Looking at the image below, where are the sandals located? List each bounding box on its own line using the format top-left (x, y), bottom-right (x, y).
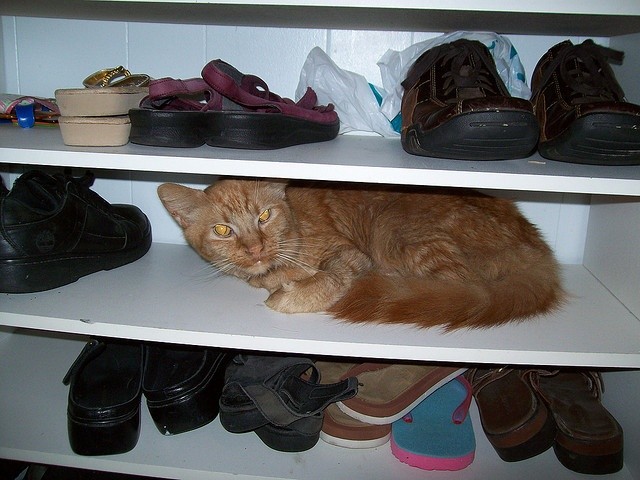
top-left (219, 351), bottom-right (358, 434)
top-left (254, 353), bottom-right (325, 453)
top-left (202, 58), bottom-right (341, 149)
top-left (128, 77), bottom-right (222, 146)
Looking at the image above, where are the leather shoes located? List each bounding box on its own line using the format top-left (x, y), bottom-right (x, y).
top-left (529, 39), bottom-right (640, 164)
top-left (399, 38), bottom-right (540, 161)
top-left (0, 169), bottom-right (152, 293)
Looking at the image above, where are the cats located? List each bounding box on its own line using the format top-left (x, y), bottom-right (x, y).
top-left (157, 173), bottom-right (581, 337)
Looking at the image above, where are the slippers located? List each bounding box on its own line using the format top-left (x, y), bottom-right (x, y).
top-left (315, 357), bottom-right (391, 449)
top-left (335, 363), bottom-right (468, 425)
top-left (390, 375), bottom-right (475, 471)
top-left (55, 65), bottom-right (154, 116)
top-left (142, 341), bottom-right (232, 437)
top-left (535, 364), bottom-right (623, 475)
top-left (467, 363), bottom-right (557, 462)
top-left (63, 335), bottom-right (150, 455)
top-left (59, 118), bottom-right (132, 148)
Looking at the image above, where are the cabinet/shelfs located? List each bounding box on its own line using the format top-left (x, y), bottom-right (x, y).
top-left (0, 0), bottom-right (640, 479)
